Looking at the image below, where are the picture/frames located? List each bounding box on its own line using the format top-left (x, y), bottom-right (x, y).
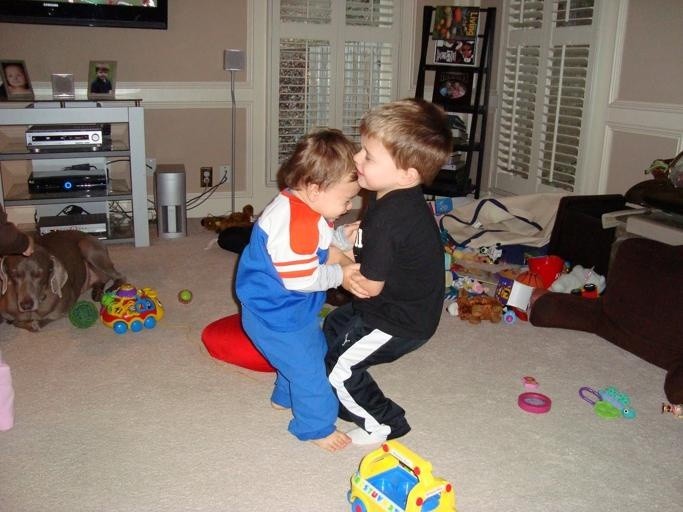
top-left (433, 38), bottom-right (479, 67)
top-left (432, 69), bottom-right (474, 108)
top-left (88, 61), bottom-right (116, 101)
top-left (0, 60), bottom-right (34, 101)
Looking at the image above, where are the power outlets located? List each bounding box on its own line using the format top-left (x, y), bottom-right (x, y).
top-left (201, 167), bottom-right (212, 187)
top-left (219, 165), bottom-right (232, 183)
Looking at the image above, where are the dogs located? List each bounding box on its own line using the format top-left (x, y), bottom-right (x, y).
top-left (0, 230), bottom-right (127, 333)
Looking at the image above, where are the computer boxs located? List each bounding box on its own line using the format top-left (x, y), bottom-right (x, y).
top-left (156, 163), bottom-right (187, 239)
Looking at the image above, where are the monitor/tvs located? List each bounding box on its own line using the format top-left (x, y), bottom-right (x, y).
top-left (0, 0), bottom-right (168, 30)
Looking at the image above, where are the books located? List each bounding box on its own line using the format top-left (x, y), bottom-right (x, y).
top-left (431, 3), bottom-right (480, 112)
top-left (448, 114), bottom-right (471, 145)
top-left (443, 154), bottom-right (466, 171)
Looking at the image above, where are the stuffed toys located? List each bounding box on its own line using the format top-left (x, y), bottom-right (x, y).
top-left (446, 291), bottom-right (505, 325)
top-left (201, 204), bottom-right (256, 233)
top-left (548, 267), bottom-right (609, 297)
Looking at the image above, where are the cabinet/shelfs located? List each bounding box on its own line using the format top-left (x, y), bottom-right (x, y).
top-left (414, 5), bottom-right (497, 199)
top-left (0, 101), bottom-right (150, 247)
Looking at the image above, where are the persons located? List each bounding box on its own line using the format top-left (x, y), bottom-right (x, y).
top-left (91, 65), bottom-right (112, 93)
top-left (5, 62), bottom-right (28, 93)
top-left (320, 98), bottom-right (452, 446)
top-left (233, 128), bottom-right (372, 452)
top-left (0, 202), bottom-right (35, 256)
